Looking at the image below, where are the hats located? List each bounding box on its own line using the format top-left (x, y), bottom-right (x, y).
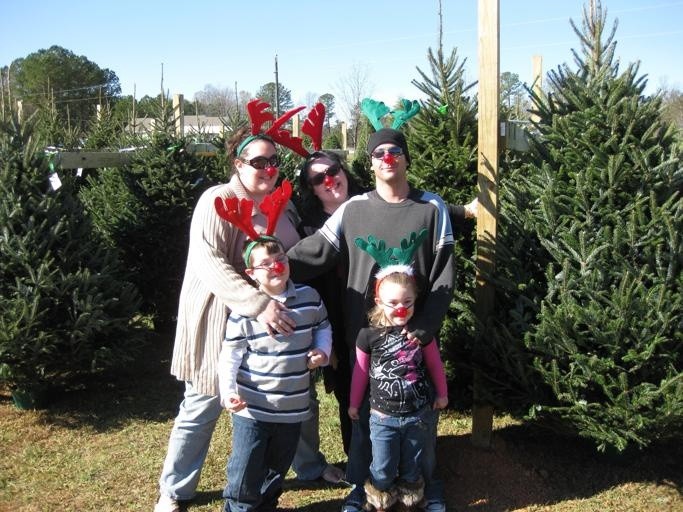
top-left (367, 127), bottom-right (410, 157)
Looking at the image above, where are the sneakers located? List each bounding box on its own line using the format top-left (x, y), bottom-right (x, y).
top-left (154, 496), bottom-right (181, 512)
top-left (341, 481), bottom-right (375, 512)
top-left (420, 487), bottom-right (446, 511)
top-left (322, 461), bottom-right (347, 480)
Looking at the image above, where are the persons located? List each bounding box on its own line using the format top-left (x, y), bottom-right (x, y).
top-left (218, 233), bottom-right (334, 512)
top-left (349, 263), bottom-right (450, 511)
top-left (287, 130), bottom-right (456, 511)
top-left (157, 127), bottom-right (346, 512)
top-left (294, 147), bottom-right (481, 457)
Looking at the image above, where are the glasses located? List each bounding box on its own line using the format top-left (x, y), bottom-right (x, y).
top-left (239, 154), bottom-right (282, 170)
top-left (371, 147), bottom-right (403, 158)
top-left (308, 162), bottom-right (342, 186)
top-left (253, 254), bottom-right (290, 270)
